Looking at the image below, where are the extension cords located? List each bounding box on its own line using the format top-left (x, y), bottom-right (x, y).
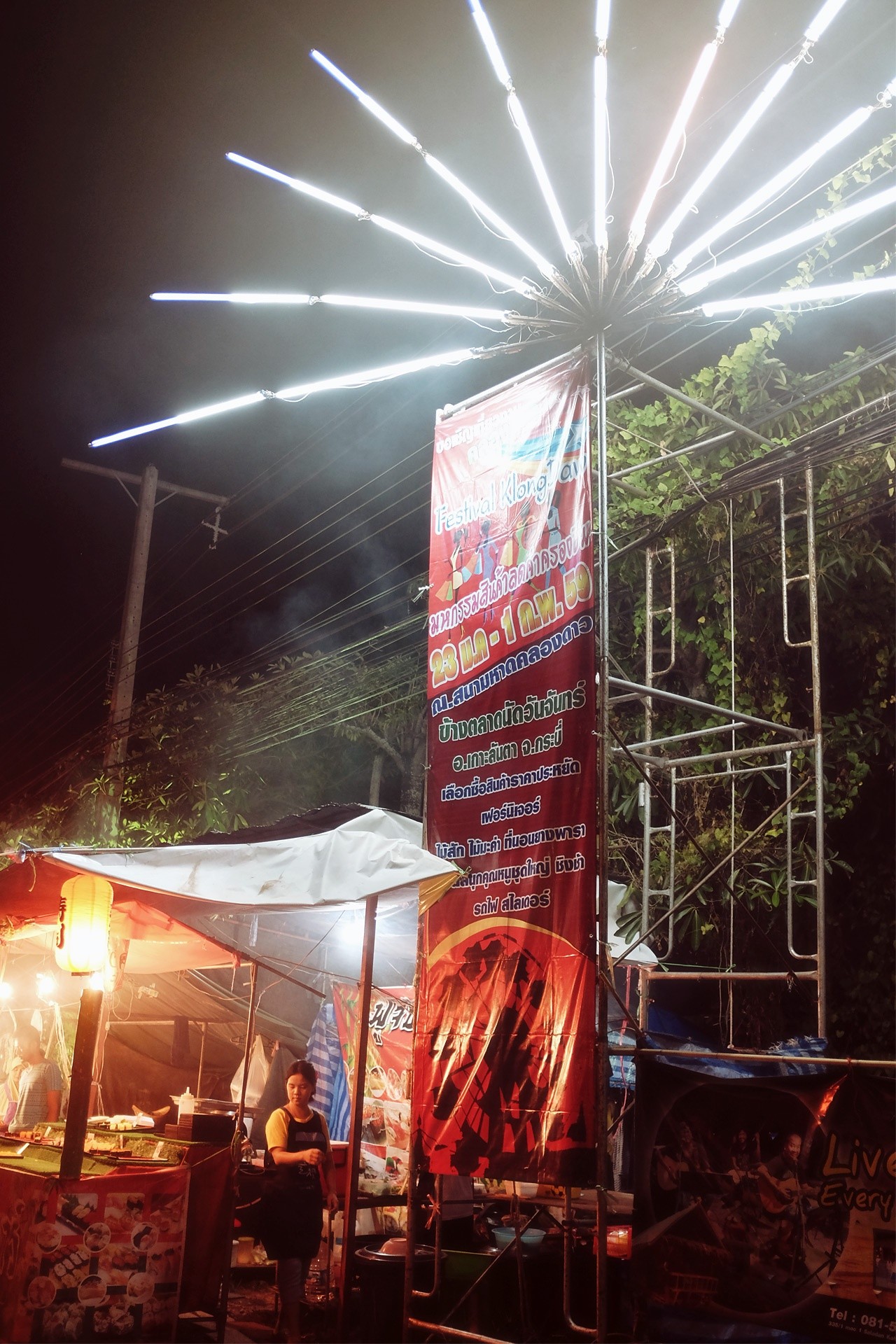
top-left (138, 986), bottom-right (159, 997)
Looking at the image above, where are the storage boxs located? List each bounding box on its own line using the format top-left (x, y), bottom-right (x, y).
top-left (318, 1140), bottom-right (349, 1199)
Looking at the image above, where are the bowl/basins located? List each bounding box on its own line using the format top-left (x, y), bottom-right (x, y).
top-left (131, 1105), bottom-right (171, 1126)
top-left (515, 1182), bottom-right (538, 1198)
top-left (378, 1237), bottom-right (407, 1255)
top-left (491, 1227), bottom-right (546, 1249)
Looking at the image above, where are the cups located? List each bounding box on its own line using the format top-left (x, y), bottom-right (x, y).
top-left (237, 1237), bottom-right (254, 1264)
top-left (230, 1240), bottom-right (239, 1268)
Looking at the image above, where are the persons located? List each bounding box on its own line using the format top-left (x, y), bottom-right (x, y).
top-left (253, 1059), bottom-right (339, 1344)
top-left (8, 1024), bottom-right (63, 1132)
top-left (637, 1064), bottom-right (821, 1308)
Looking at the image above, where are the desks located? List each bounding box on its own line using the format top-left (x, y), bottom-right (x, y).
top-left (476, 1184), bottom-right (635, 1344)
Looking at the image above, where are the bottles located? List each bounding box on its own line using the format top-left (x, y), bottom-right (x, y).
top-left (304, 1241), bottom-right (336, 1304)
top-left (177, 1086), bottom-right (195, 1125)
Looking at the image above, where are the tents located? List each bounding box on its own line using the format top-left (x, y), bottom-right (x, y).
top-left (0, 803), bottom-right (656, 1344)
top-left (304, 1006), bottom-right (830, 1207)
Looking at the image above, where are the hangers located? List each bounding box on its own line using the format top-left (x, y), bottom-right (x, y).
top-left (230, 1021), bottom-right (280, 1048)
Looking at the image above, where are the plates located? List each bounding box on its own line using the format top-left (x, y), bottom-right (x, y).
top-left (77, 1274), bottom-right (107, 1307)
top-left (343, 1060), bottom-right (406, 1101)
top-left (126, 1272), bottom-right (154, 1304)
top-left (131, 1221), bottom-right (159, 1252)
top-left (27, 1276), bottom-right (57, 1309)
top-left (83, 1223), bottom-right (111, 1253)
top-left (35, 1222), bottom-right (62, 1254)
